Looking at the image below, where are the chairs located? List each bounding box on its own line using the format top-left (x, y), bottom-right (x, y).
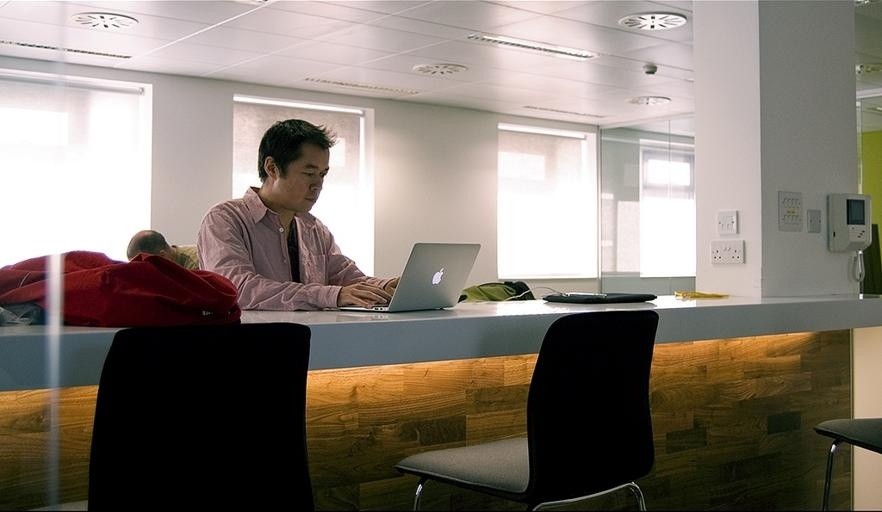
top-left (813, 418), bottom-right (882, 512)
top-left (88, 322), bottom-right (315, 512)
top-left (395, 310), bottom-right (659, 512)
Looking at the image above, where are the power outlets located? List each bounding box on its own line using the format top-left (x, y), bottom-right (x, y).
top-left (710, 239), bottom-right (726, 264)
top-left (726, 240), bottom-right (745, 266)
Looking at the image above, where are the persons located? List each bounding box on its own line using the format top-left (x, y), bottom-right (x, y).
top-left (197, 118), bottom-right (401, 313)
top-left (127, 230), bottom-right (201, 271)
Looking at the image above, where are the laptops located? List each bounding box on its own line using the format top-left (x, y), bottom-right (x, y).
top-left (338, 244), bottom-right (482, 311)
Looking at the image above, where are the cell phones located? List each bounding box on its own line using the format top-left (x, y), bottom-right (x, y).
top-left (565, 293), bottom-right (608, 297)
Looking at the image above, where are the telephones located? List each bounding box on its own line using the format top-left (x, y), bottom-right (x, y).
top-left (828, 193), bottom-right (872, 253)
